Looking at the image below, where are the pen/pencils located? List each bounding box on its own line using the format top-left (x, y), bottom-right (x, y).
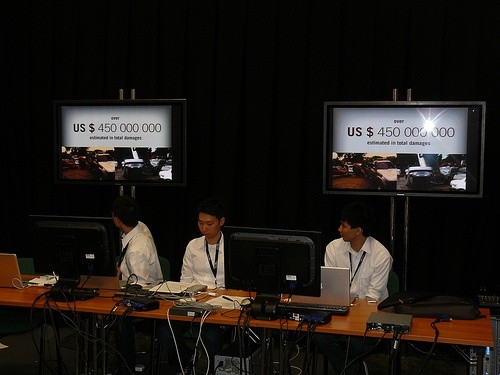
top-left (222, 295), bottom-right (234, 301)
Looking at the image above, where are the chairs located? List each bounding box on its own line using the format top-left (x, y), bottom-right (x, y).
top-left (117, 257), bottom-right (170, 375)
top-left (324, 272), bottom-right (403, 375)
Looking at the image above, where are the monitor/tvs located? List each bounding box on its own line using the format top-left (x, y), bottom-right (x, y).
top-left (223, 226), bottom-right (322, 320)
top-left (50, 99), bottom-right (187, 187)
top-left (27, 214), bottom-right (120, 300)
top-left (322, 101), bottom-right (486, 198)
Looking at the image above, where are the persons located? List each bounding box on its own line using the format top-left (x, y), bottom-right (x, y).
top-left (398, 175), bottom-right (408, 190)
top-left (312, 202), bottom-right (393, 375)
top-left (78, 197), bottom-right (164, 375)
top-left (115, 167), bottom-right (124, 180)
top-left (168, 204), bottom-right (226, 375)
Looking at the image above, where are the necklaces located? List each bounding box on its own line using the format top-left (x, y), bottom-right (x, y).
top-left (116, 233), bottom-right (130, 270)
top-left (204, 237), bottom-right (221, 284)
top-left (348, 251), bottom-right (366, 287)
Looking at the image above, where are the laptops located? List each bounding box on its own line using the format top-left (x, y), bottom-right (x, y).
top-left (0, 253), bottom-right (34, 288)
top-left (79, 262), bottom-right (126, 290)
top-left (291, 267), bottom-right (358, 306)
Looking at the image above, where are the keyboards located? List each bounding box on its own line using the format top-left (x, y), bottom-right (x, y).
top-left (281, 302), bottom-right (349, 315)
top-left (377, 292), bottom-right (422, 311)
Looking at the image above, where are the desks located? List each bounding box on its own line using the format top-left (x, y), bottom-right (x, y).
top-left (0, 273), bottom-right (493, 375)
